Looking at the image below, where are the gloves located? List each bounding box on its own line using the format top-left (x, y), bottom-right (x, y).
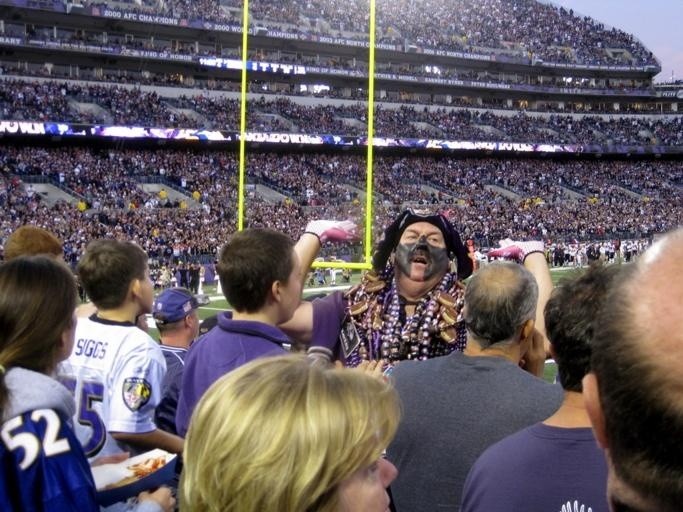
top-left (305, 219), bottom-right (358, 247)
top-left (486, 238), bottom-right (545, 264)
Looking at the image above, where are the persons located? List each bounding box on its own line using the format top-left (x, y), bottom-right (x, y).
top-left (378, 259), bottom-right (566, 512)
top-left (73, 298), bottom-right (149, 335)
top-left (1, 255), bottom-right (176, 510)
top-left (178, 355), bottom-right (403, 510)
top-left (176, 226), bottom-right (304, 438)
top-left (49, 238), bottom-right (185, 454)
top-left (1, 1), bottom-right (681, 296)
top-left (460, 262), bottom-right (618, 511)
top-left (151, 286), bottom-right (200, 436)
top-left (580, 224), bottom-right (681, 511)
top-left (2, 225), bottom-right (65, 264)
top-left (275, 208), bottom-right (555, 382)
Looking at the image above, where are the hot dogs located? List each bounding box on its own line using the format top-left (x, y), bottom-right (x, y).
top-left (105, 456), bottom-right (166, 490)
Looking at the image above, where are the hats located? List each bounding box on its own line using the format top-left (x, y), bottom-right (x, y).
top-left (151, 287), bottom-right (210, 324)
